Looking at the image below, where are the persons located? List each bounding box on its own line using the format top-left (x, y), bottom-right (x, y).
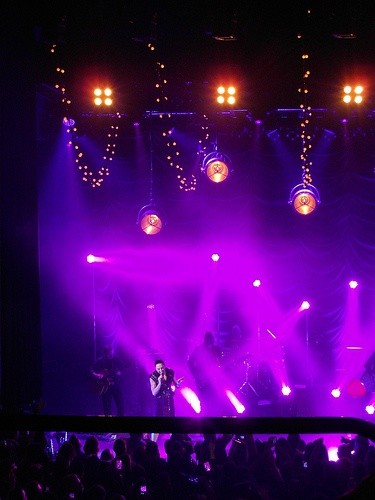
top-left (188, 332), bottom-right (227, 416)
top-left (148, 360), bottom-right (186, 417)
top-left (0, 428), bottom-right (375, 500)
top-left (241, 351), bottom-right (279, 411)
top-left (90, 346), bottom-right (125, 416)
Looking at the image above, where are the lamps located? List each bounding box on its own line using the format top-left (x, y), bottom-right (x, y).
top-left (288, 184), bottom-right (321, 216)
top-left (201, 151), bottom-right (234, 182)
top-left (136, 204), bottom-right (165, 237)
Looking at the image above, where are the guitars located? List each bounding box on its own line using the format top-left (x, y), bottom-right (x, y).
top-left (93, 362), bottom-right (129, 396)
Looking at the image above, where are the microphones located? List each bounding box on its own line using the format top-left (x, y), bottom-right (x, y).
top-left (160, 372), bottom-right (164, 383)
